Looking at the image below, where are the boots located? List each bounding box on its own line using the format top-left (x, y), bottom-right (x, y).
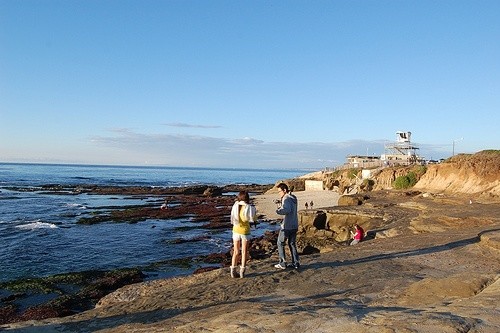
top-left (229, 266), bottom-right (238, 278)
top-left (239, 266), bottom-right (246, 278)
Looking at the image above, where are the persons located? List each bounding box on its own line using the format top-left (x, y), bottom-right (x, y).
top-left (230, 191), bottom-right (258, 279)
top-left (349, 224), bottom-right (363, 246)
top-left (310, 201), bottom-right (314, 210)
top-left (160, 199), bottom-right (171, 212)
top-left (273, 183), bottom-right (301, 271)
top-left (305, 202), bottom-right (308, 210)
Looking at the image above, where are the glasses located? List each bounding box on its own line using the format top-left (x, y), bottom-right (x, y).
top-left (278, 190), bottom-right (282, 193)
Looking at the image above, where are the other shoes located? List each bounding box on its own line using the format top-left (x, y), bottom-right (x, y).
top-left (275, 264), bottom-right (286, 270)
top-left (287, 264), bottom-right (298, 269)
